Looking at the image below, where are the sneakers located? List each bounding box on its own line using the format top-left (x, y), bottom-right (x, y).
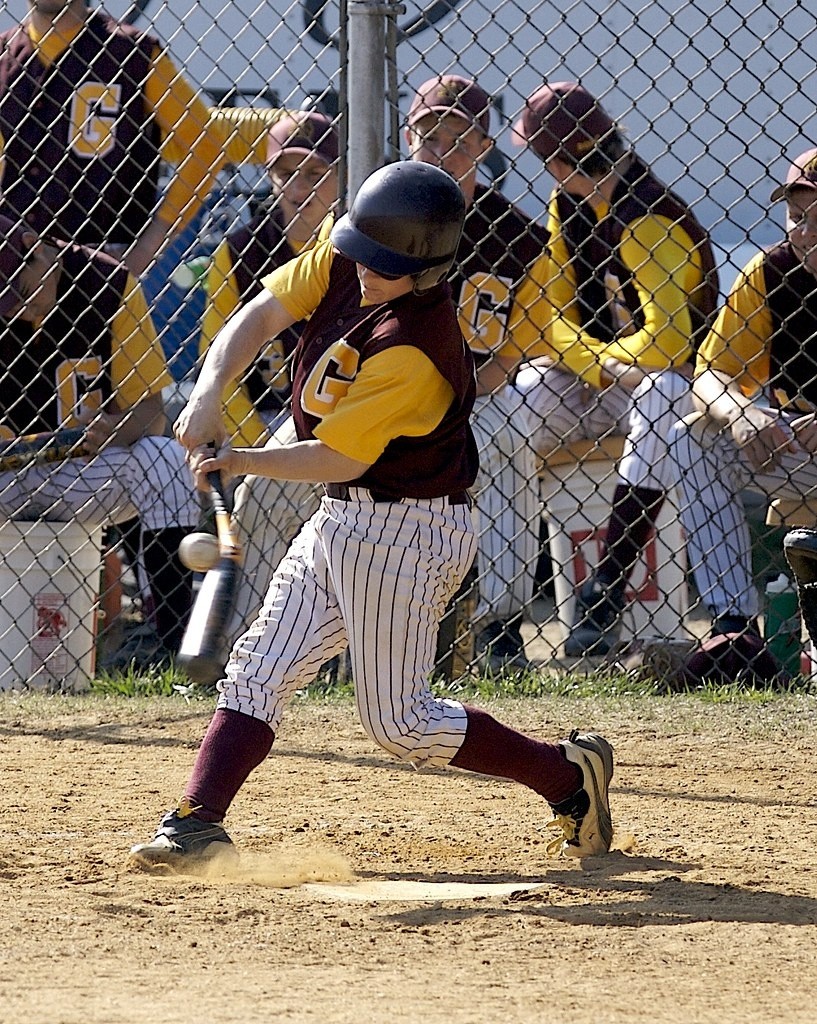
top-left (129, 795), bottom-right (240, 876)
top-left (564, 577), bottom-right (626, 656)
top-left (471, 611), bottom-right (539, 684)
top-left (538, 729), bottom-right (613, 858)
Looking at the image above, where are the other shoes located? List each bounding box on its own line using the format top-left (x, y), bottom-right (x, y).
top-left (102, 624), bottom-right (171, 679)
top-left (295, 655), bottom-right (340, 697)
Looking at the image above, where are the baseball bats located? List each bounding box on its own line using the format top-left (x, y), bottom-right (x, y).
top-left (177, 443), bottom-right (243, 686)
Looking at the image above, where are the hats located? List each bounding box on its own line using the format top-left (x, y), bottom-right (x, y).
top-left (0, 215), bottom-right (26, 317)
top-left (263, 113), bottom-right (339, 166)
top-left (770, 148), bottom-right (817, 204)
top-left (512, 81), bottom-right (613, 157)
top-left (406, 75), bottom-right (490, 136)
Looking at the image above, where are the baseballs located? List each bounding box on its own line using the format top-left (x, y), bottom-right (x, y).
top-left (177, 532), bottom-right (220, 573)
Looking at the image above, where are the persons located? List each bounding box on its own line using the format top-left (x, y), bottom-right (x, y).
top-left (0, 0), bottom-right (228, 283)
top-left (661, 147), bottom-right (817, 692)
top-left (508, 80), bottom-right (720, 655)
top-left (127, 162), bottom-right (616, 882)
top-left (199, 112), bottom-right (343, 529)
top-left (214, 74), bottom-right (553, 681)
top-left (0, 215), bottom-right (199, 681)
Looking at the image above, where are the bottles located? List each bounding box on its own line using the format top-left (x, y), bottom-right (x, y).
top-left (173, 256), bottom-right (211, 289)
top-left (762, 574), bottom-right (803, 690)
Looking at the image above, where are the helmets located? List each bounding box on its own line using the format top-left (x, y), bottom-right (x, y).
top-left (330, 160), bottom-right (466, 291)
